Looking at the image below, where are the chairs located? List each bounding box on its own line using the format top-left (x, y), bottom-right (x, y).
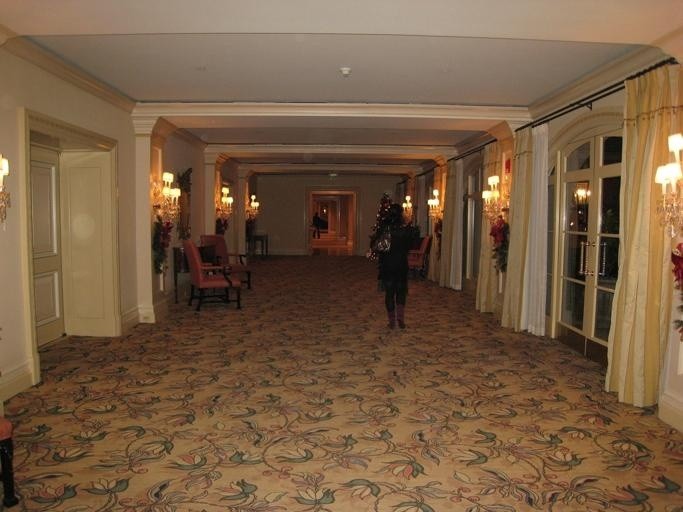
top-left (404, 236), bottom-right (430, 278)
top-left (184, 232), bottom-right (254, 311)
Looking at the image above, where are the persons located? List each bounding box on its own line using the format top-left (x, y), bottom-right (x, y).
top-left (370, 204), bottom-right (424, 329)
top-left (313, 212), bottom-right (320, 239)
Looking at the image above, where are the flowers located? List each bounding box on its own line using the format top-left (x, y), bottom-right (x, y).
top-left (489, 220), bottom-right (511, 272)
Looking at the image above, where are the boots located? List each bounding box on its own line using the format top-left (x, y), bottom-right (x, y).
top-left (386, 309), bottom-right (395, 330)
top-left (396, 304), bottom-right (406, 329)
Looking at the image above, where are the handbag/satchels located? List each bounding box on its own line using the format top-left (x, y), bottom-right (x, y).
top-left (371, 231), bottom-right (391, 252)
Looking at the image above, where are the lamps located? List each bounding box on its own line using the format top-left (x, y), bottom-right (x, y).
top-left (655, 132), bottom-right (683, 242)
top-left (428, 190), bottom-right (444, 222)
top-left (245, 194), bottom-right (261, 221)
top-left (158, 172), bottom-right (183, 222)
top-left (477, 174), bottom-right (513, 224)
top-left (216, 186), bottom-right (232, 229)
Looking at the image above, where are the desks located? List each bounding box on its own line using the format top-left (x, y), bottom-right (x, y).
top-left (174, 245), bottom-right (218, 304)
top-left (247, 232), bottom-right (270, 261)
